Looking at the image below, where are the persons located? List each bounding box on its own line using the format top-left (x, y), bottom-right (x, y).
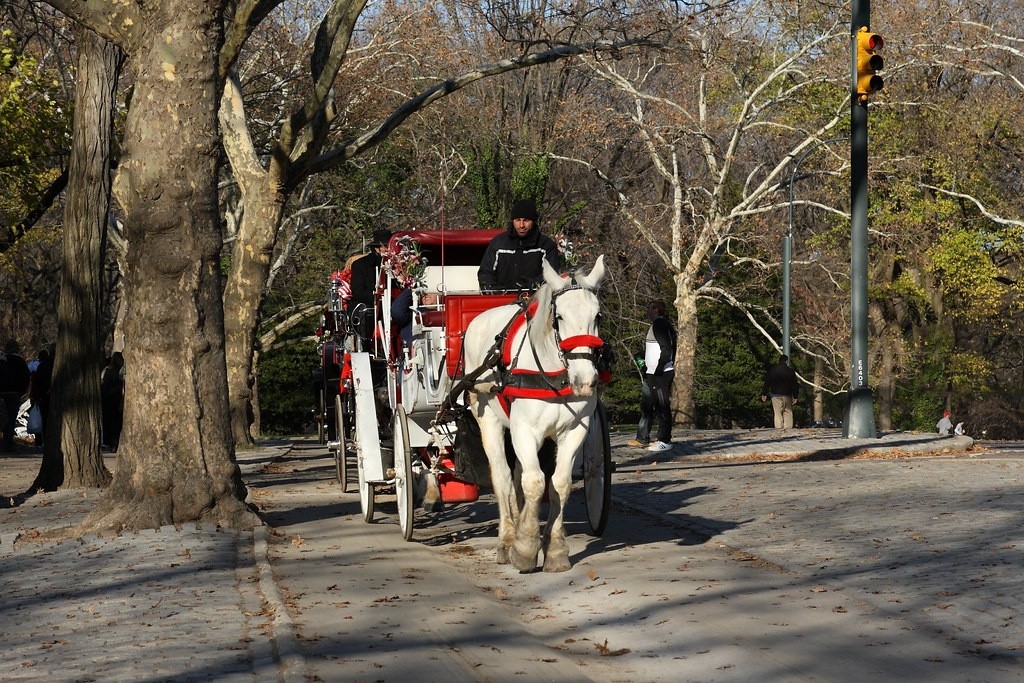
top-left (477, 200), bottom-right (566, 295)
top-left (391, 284), bottom-right (443, 384)
top-left (627, 300), bottom-right (677, 452)
top-left (762, 354), bottom-right (798, 429)
top-left (346, 229), bottom-right (392, 340)
top-left (0, 339), bottom-right (124, 454)
top-left (936, 411), bottom-right (965, 436)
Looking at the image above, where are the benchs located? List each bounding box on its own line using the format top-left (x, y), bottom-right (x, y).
top-left (408, 265), bottom-right (485, 382)
top-left (317, 300), bottom-right (397, 435)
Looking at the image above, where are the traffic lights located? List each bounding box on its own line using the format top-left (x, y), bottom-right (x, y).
top-left (850, 25), bottom-right (884, 105)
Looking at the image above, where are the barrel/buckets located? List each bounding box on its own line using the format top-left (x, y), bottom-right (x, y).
top-left (437, 455), bottom-right (478, 503)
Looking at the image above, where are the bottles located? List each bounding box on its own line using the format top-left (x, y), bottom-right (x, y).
top-left (636, 357), bottom-right (647, 373)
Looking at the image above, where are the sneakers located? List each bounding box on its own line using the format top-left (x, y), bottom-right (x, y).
top-left (648, 441), bottom-right (673, 452)
top-left (627, 440), bottom-right (649, 447)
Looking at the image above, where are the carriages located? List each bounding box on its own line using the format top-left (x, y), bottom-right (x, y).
top-left (313, 229), bottom-right (618, 576)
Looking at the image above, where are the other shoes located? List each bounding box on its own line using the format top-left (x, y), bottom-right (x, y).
top-left (2, 441), bottom-right (15, 447)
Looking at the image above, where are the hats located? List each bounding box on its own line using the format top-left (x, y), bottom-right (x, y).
top-left (368, 230), bottom-right (392, 246)
top-left (510, 200), bottom-right (538, 222)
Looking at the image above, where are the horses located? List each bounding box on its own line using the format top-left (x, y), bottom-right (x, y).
top-left (422, 454), bottom-right (446, 511)
top-left (463, 255), bottom-right (606, 573)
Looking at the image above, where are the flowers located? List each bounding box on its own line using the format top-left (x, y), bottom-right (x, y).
top-left (379, 235), bottom-right (428, 289)
